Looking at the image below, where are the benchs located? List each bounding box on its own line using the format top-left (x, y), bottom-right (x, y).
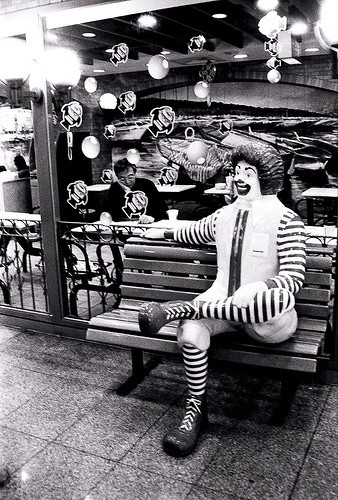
top-left (86, 236), bottom-right (334, 426)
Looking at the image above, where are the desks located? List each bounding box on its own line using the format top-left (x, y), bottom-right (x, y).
top-left (88, 183), bottom-right (196, 209)
top-left (302, 186), bottom-right (338, 226)
top-left (204, 185), bottom-right (284, 197)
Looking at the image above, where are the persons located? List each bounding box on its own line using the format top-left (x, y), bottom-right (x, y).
top-left (97, 158), bottom-right (170, 224)
top-left (138, 141), bottom-right (307, 458)
top-left (14, 154), bottom-right (30, 178)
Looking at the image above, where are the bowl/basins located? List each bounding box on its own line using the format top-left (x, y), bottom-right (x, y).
top-left (215, 183), bottom-right (226, 190)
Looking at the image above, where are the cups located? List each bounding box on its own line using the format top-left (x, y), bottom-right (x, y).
top-left (225, 176), bottom-right (233, 187)
top-left (167, 209), bottom-right (178, 220)
